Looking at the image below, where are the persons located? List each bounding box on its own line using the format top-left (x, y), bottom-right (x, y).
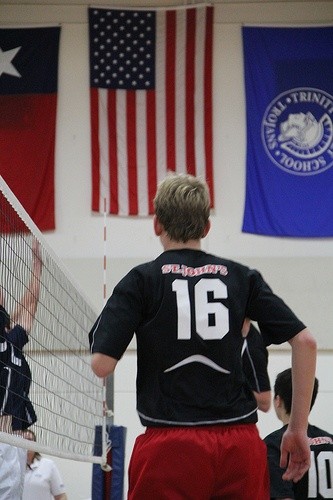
top-left (263, 368), bottom-right (333, 500)
top-left (88, 174), bottom-right (316, 500)
top-left (241, 319), bottom-right (272, 413)
top-left (21, 430), bottom-right (67, 500)
top-left (0, 236), bottom-right (42, 500)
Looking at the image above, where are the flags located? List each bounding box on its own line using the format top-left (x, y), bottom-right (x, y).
top-left (0, 22), bottom-right (61, 235)
top-left (241, 23), bottom-right (333, 240)
top-left (88, 4), bottom-right (216, 216)
